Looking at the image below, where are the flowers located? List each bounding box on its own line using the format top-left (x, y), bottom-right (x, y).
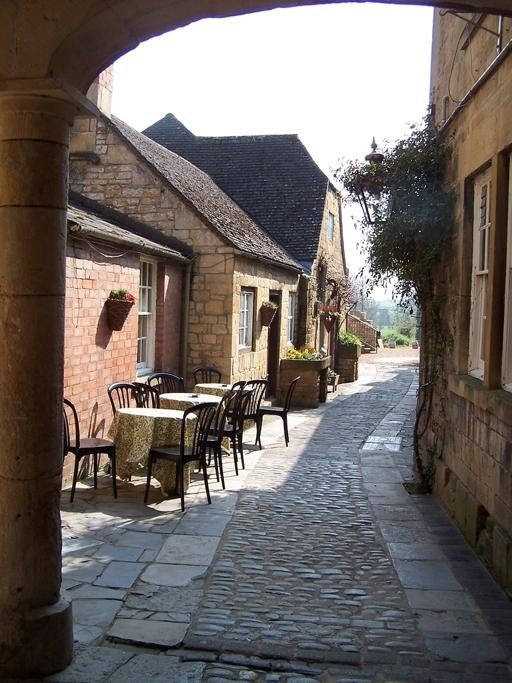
top-left (109, 287), bottom-right (135, 302)
top-left (262, 299), bottom-right (278, 309)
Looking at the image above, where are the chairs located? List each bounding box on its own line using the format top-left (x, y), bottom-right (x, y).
top-left (62, 366), bottom-right (305, 512)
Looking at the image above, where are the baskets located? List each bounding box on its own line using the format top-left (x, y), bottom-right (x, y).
top-left (106, 298), bottom-right (134, 330)
top-left (261, 307), bottom-right (277, 326)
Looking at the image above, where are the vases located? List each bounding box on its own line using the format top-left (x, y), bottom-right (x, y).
top-left (260, 306), bottom-right (279, 327)
top-left (102, 298), bottom-right (136, 332)
